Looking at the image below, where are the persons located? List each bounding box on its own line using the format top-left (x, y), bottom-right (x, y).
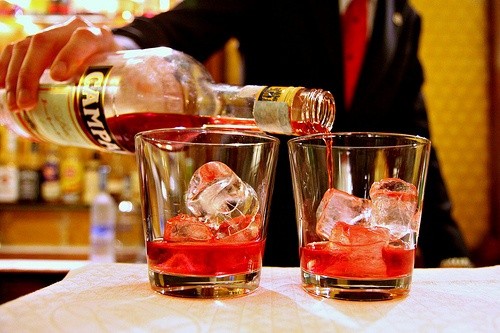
top-left (0, 0), bottom-right (474, 267)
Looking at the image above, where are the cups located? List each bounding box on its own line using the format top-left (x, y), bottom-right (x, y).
top-left (134, 128), bottom-right (279, 300)
top-left (287, 132), bottom-right (431, 301)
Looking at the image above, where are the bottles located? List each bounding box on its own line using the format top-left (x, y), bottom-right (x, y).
top-left (0, 45), bottom-right (336, 154)
top-left (0, 133), bottom-right (102, 206)
top-left (88, 166), bottom-right (116, 264)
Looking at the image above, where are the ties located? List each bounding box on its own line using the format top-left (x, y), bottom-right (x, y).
top-left (341, 0), bottom-right (368, 111)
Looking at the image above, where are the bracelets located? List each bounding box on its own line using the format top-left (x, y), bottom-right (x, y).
top-left (438, 259), bottom-right (470, 266)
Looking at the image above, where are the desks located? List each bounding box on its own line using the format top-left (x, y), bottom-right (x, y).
top-left (0, 263), bottom-right (500, 333)
